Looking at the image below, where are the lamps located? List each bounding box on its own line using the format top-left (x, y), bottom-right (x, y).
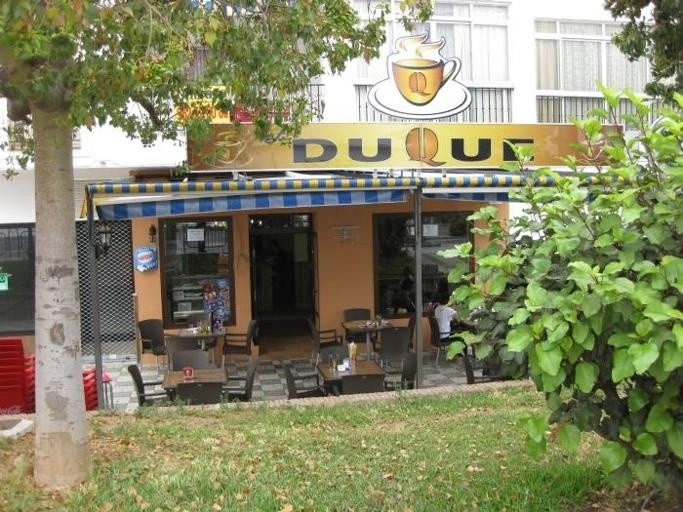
top-left (94, 221), bottom-right (117, 257)
top-left (405, 219), bottom-right (416, 242)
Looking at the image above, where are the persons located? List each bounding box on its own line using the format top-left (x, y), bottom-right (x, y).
top-left (433, 293), bottom-right (469, 357)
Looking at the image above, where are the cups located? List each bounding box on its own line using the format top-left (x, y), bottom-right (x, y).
top-left (337, 358), bottom-right (350, 371)
top-left (392, 57), bottom-right (461, 105)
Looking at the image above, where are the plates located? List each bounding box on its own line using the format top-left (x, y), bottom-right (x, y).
top-left (367, 77), bottom-right (472, 120)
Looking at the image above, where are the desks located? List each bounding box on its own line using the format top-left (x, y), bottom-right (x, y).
top-left (161, 326), bottom-right (227, 361)
top-left (161, 368), bottom-right (230, 404)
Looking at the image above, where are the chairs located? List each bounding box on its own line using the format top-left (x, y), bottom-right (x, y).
top-left (222, 345), bottom-right (261, 402)
top-left (220, 319), bottom-right (259, 370)
top-left (147, 323), bottom-right (174, 373)
top-left (163, 334), bottom-right (205, 353)
top-left (136, 319), bottom-right (165, 355)
top-left (169, 349), bottom-right (209, 372)
top-left (127, 363), bottom-right (174, 406)
top-left (275, 307), bottom-right (490, 397)
top-left (0, 336), bottom-right (113, 414)
top-left (187, 312), bottom-right (208, 324)
top-left (176, 379), bottom-right (223, 405)
